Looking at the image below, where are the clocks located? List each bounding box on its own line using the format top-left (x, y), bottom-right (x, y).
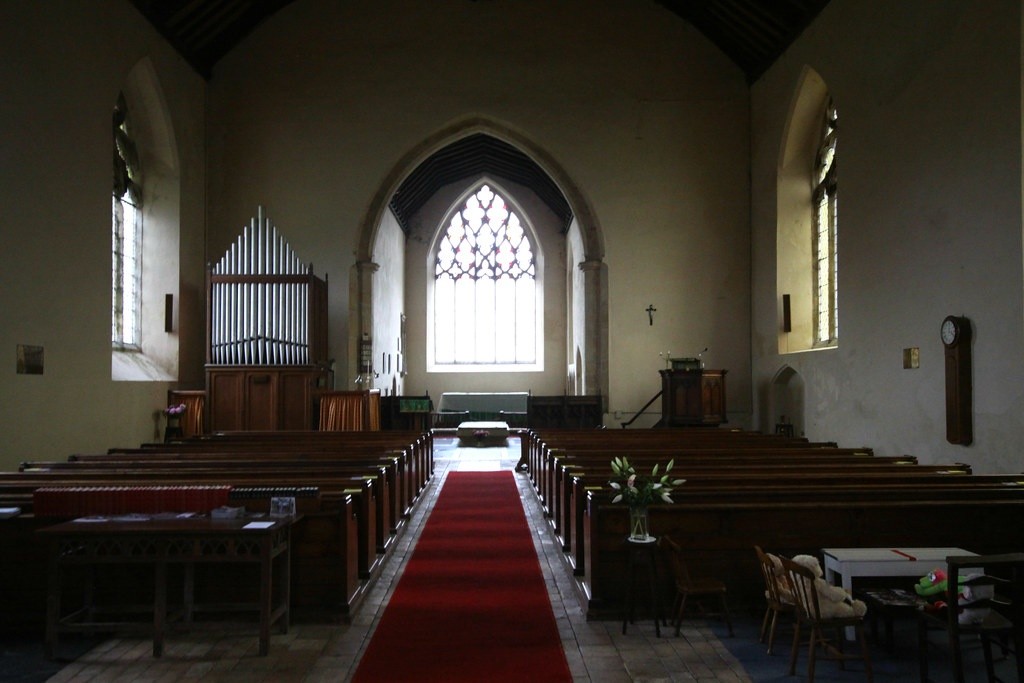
top-left (941, 316), bottom-right (971, 348)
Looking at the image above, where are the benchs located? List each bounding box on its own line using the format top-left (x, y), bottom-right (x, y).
top-left (515, 421), bottom-right (1023, 621)
top-left (0, 433), bottom-right (432, 626)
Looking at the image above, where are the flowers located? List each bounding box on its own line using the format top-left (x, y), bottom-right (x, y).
top-left (162, 402), bottom-right (186, 418)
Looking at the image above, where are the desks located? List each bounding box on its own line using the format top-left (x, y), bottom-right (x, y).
top-left (818, 547), bottom-right (987, 649)
top-left (32, 513), bottom-right (294, 660)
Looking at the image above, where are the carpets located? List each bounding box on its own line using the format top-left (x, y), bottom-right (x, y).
top-left (350, 471), bottom-right (575, 683)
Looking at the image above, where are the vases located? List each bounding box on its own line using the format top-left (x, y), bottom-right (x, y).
top-left (169, 419), bottom-right (180, 428)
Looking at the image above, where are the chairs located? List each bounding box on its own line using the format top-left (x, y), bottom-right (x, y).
top-left (915, 554), bottom-right (1023, 682)
top-left (780, 551), bottom-right (876, 683)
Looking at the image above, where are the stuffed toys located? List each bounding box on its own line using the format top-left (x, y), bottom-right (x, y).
top-left (790, 552), bottom-right (868, 620)
top-left (914, 568), bottom-right (971, 606)
top-left (955, 573), bottom-right (992, 627)
top-left (761, 551), bottom-right (797, 607)
